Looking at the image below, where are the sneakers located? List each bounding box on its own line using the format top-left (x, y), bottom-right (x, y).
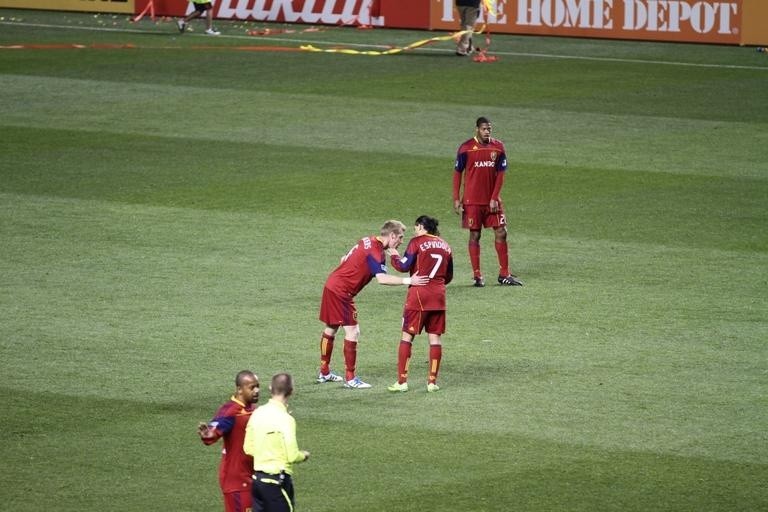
top-left (473, 276), bottom-right (485, 287)
top-left (317, 372), bottom-right (343, 383)
top-left (456, 48), bottom-right (469, 56)
top-left (470, 46), bottom-right (475, 53)
top-left (204, 28), bottom-right (221, 36)
top-left (344, 376), bottom-right (372, 389)
top-left (388, 381), bottom-right (408, 392)
top-left (176, 18), bottom-right (186, 33)
top-left (427, 383), bottom-right (439, 392)
top-left (498, 274), bottom-right (523, 287)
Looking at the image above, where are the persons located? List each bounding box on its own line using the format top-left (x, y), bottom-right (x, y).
top-left (243, 373), bottom-right (310, 512)
top-left (176, 0), bottom-right (221, 35)
top-left (386, 216), bottom-right (453, 393)
top-left (436, 0), bottom-right (508, 57)
top-left (316, 219), bottom-right (430, 390)
top-left (452, 117), bottom-right (524, 287)
top-left (197, 370), bottom-right (260, 512)
